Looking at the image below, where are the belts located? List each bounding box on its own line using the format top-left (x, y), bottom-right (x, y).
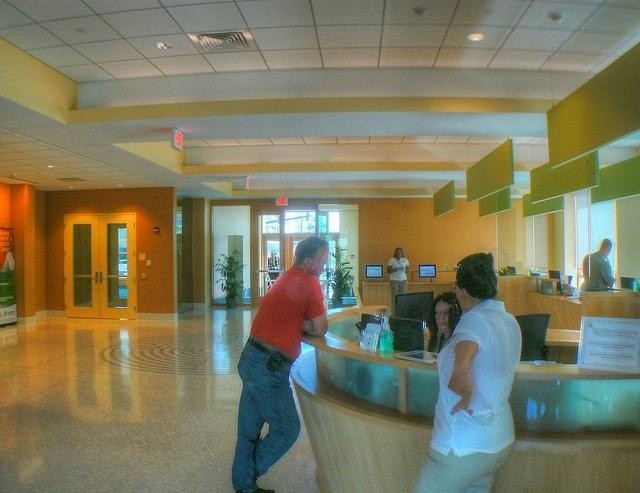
top-left (248, 339), bottom-right (293, 363)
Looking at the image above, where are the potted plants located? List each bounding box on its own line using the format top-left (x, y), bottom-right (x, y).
top-left (215, 250), bottom-right (248, 309)
top-left (325, 244), bottom-right (356, 309)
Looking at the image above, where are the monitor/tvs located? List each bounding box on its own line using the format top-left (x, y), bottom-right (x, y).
top-left (419, 265), bottom-right (436, 278)
top-left (620, 277), bottom-right (640, 290)
top-left (507, 265), bottom-right (516, 273)
top-left (361, 314), bottom-right (423, 351)
top-left (366, 264), bottom-right (383, 279)
top-left (549, 269), bottom-right (561, 292)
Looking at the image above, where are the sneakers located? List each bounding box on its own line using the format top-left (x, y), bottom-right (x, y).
top-left (236, 488), bottom-right (274, 493)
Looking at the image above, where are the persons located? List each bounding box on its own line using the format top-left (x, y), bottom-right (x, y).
top-left (409, 249), bottom-right (524, 492)
top-left (578, 239), bottom-right (616, 294)
top-left (425, 294), bottom-right (463, 356)
top-left (386, 247), bottom-right (411, 316)
top-left (230, 235), bottom-right (329, 493)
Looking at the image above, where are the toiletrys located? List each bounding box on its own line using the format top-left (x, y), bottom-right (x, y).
top-left (379, 316), bottom-right (395, 353)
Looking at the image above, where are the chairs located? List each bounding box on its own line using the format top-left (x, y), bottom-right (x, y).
top-left (513, 313), bottom-right (553, 363)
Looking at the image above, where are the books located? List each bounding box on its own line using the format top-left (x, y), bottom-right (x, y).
top-left (362, 322), bottom-right (380, 351)
top-left (395, 348), bottom-right (441, 366)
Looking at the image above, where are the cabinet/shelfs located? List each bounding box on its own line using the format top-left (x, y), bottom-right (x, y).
top-left (360, 266), bottom-right (640, 333)
